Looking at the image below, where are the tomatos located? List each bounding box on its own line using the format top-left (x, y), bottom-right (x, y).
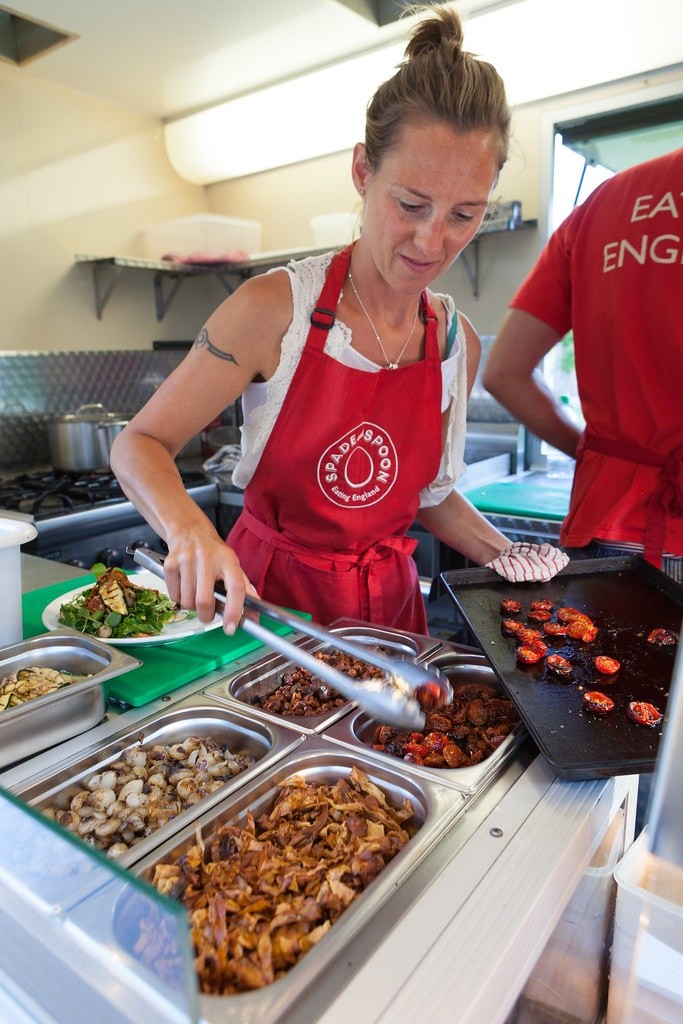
top-left (372, 682), bottom-right (451, 768)
top-left (499, 598), bottom-right (679, 725)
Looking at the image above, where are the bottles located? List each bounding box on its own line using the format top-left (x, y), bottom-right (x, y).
top-left (547, 397), bottom-right (583, 478)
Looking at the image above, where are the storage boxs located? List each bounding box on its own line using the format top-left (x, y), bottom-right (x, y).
top-left (146, 214), bottom-right (261, 266)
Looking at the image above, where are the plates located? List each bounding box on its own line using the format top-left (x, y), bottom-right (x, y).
top-left (42, 573), bottom-right (224, 648)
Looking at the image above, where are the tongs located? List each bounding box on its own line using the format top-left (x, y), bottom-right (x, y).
top-left (124, 546), bottom-right (454, 730)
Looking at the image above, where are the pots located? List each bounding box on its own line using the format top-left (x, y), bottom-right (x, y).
top-left (47, 403), bottom-right (137, 472)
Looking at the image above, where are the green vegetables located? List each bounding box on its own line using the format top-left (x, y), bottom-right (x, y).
top-left (59, 563), bottom-right (198, 638)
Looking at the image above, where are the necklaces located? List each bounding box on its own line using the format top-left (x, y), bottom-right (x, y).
top-left (346, 265), bottom-right (419, 369)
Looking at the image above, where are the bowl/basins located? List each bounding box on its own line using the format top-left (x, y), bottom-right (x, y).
top-left (312, 211), bottom-right (362, 247)
top-left (150, 213), bottom-right (261, 263)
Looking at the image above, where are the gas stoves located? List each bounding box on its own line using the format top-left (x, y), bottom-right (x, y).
top-left (0, 463), bottom-right (219, 571)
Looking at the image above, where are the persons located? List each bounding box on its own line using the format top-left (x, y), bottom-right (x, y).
top-left (480, 145), bottom-right (683, 568)
top-left (110, 5), bottom-right (515, 635)
